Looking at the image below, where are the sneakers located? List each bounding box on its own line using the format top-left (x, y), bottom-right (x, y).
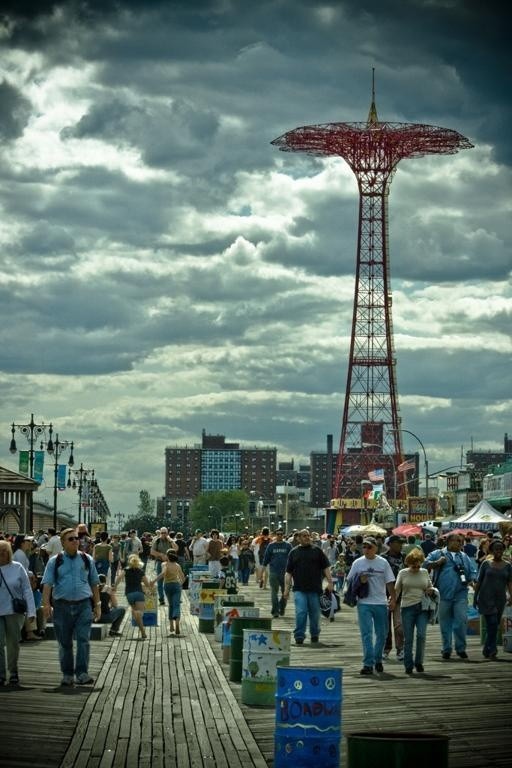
top-left (360, 661), bottom-right (383, 674)
top-left (170, 623), bottom-right (180, 634)
top-left (272, 608), bottom-right (284, 618)
top-left (443, 652), bottom-right (450, 659)
top-left (160, 601), bottom-right (165, 605)
top-left (141, 628), bottom-right (147, 638)
top-left (296, 637), bottom-right (303, 644)
top-left (109, 630), bottom-right (122, 636)
top-left (405, 662), bottom-right (424, 674)
top-left (180, 600), bottom-right (182, 603)
top-left (382, 650), bottom-right (404, 661)
top-left (132, 610), bottom-right (141, 624)
top-left (483, 646), bottom-right (498, 659)
top-left (1, 676), bottom-right (20, 692)
top-left (456, 651), bottom-right (467, 658)
top-left (62, 673), bottom-right (94, 686)
top-left (311, 635), bottom-right (318, 642)
top-left (260, 579), bottom-right (267, 589)
top-left (26, 629), bottom-right (45, 641)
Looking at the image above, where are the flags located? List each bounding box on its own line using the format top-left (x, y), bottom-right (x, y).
top-left (397, 457), bottom-right (416, 473)
top-left (367, 468), bottom-right (385, 482)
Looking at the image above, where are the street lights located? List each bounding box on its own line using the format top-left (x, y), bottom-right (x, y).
top-left (385, 427), bottom-right (449, 523)
top-left (39, 428), bottom-right (128, 537)
top-left (6, 412), bottom-right (56, 535)
top-left (360, 442), bottom-right (477, 508)
top-left (206, 478), bottom-right (319, 540)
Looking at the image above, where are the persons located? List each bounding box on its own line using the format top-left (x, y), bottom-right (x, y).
top-left (1, 523), bottom-right (511, 697)
top-left (376, 490), bottom-right (394, 511)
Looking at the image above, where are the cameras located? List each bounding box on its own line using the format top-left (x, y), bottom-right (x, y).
top-left (454, 563), bottom-right (468, 586)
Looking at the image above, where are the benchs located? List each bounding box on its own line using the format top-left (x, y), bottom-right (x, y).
top-left (41, 622), bottom-right (109, 640)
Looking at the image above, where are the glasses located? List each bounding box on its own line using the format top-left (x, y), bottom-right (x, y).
top-left (63, 536), bottom-right (79, 542)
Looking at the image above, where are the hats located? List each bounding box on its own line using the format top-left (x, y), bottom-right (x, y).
top-left (386, 536), bottom-right (406, 545)
top-left (363, 537), bottom-right (378, 548)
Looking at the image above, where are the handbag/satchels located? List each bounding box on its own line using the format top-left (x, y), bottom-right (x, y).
top-left (13, 599), bottom-right (27, 613)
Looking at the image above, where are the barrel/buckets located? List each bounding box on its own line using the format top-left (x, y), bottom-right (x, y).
top-left (244, 629), bottom-right (290, 707)
top-left (274, 664), bottom-right (344, 763)
top-left (187, 561), bottom-right (256, 658)
top-left (228, 616), bottom-right (273, 681)
